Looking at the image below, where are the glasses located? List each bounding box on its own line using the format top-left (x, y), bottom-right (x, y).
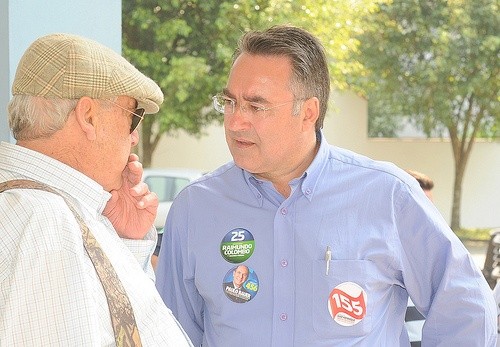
top-left (105, 99), bottom-right (145, 134)
top-left (212, 94), bottom-right (310, 123)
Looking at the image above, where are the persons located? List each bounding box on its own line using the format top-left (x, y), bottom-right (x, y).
top-left (0, 35), bottom-right (195, 347)
top-left (224, 265), bottom-right (250, 302)
top-left (152, 23), bottom-right (497, 347)
top-left (396, 168), bottom-right (435, 347)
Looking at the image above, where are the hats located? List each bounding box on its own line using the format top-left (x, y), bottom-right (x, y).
top-left (10, 34), bottom-right (164, 114)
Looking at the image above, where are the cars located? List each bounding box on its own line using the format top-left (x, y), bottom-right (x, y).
top-left (141, 168), bottom-right (210, 235)
